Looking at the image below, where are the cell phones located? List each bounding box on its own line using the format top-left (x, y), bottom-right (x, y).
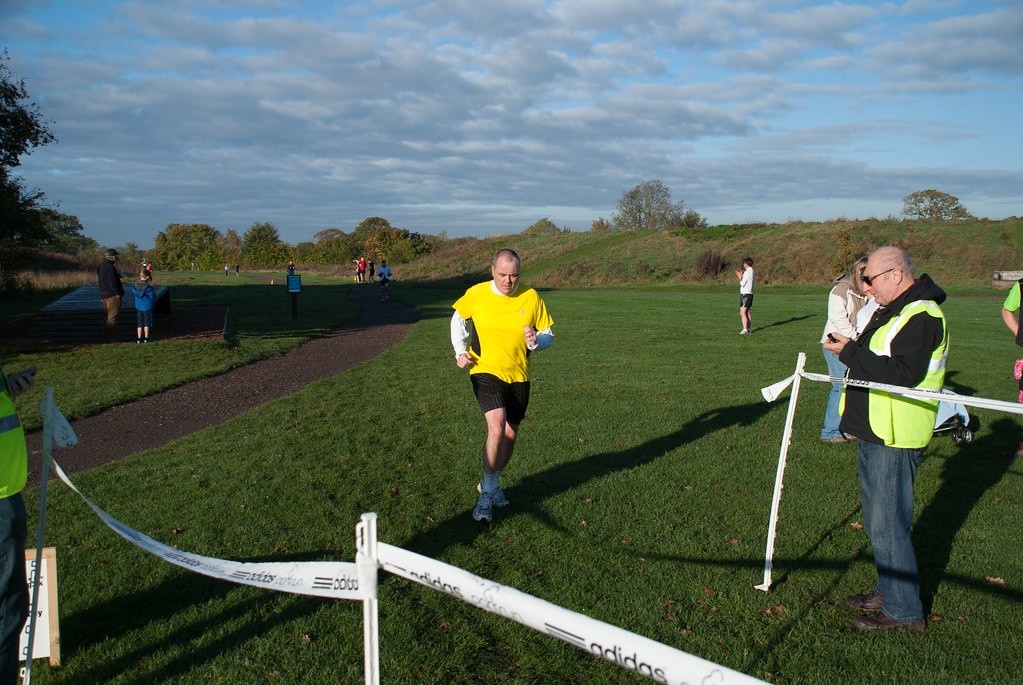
top-left (15, 368), bottom-right (37, 383)
top-left (827, 333), bottom-right (839, 343)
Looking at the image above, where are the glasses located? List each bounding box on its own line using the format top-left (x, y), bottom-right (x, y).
top-left (862, 269), bottom-right (903, 286)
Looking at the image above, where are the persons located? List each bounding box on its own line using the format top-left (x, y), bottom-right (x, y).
top-left (450, 248), bottom-right (556, 523)
top-left (0, 363), bottom-right (36, 685)
top-left (823, 245), bottom-right (951, 631)
top-left (287, 260), bottom-right (296, 275)
top-left (820, 255), bottom-right (881, 444)
top-left (235, 263), bottom-right (240, 276)
top-left (1002, 278), bottom-right (1023, 457)
top-left (353, 258), bottom-right (375, 284)
top-left (133, 269), bottom-right (156, 343)
top-left (376, 260), bottom-right (392, 301)
top-left (225, 263), bottom-right (230, 276)
top-left (736, 257), bottom-right (754, 335)
top-left (96, 249), bottom-right (124, 343)
top-left (146, 262), bottom-right (152, 274)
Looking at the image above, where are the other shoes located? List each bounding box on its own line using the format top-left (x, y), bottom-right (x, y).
top-left (842, 431), bottom-right (855, 439)
top-left (739, 329), bottom-right (751, 335)
top-left (382, 296), bottom-right (389, 302)
top-left (144, 339), bottom-right (149, 343)
top-left (821, 433), bottom-right (847, 443)
top-left (136, 338), bottom-right (142, 343)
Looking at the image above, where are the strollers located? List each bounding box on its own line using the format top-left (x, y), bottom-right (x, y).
top-left (932, 389), bottom-right (974, 446)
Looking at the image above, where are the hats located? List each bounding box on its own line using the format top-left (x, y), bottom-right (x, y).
top-left (105, 249), bottom-right (120, 256)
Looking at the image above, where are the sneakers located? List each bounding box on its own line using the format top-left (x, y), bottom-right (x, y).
top-left (845, 591), bottom-right (884, 611)
top-left (477, 480), bottom-right (510, 508)
top-left (472, 489), bottom-right (493, 522)
top-left (854, 611), bottom-right (926, 631)
